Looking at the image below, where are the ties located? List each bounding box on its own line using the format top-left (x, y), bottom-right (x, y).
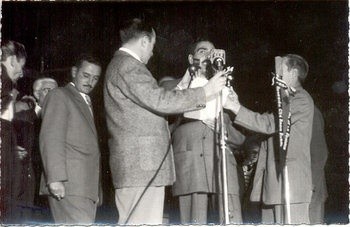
top-left (86, 96), bottom-right (95, 111)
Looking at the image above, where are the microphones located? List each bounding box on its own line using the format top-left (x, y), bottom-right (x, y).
top-left (273, 56), bottom-right (286, 89)
top-left (213, 57), bottom-right (233, 81)
top-left (188, 64), bottom-right (201, 74)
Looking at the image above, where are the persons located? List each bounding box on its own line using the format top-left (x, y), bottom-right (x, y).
top-left (158, 38), bottom-right (248, 224)
top-left (15, 76), bottom-right (58, 226)
top-left (37, 52), bottom-right (106, 227)
top-left (156, 74), bottom-right (183, 226)
top-left (308, 101), bottom-right (329, 224)
top-left (0, 39), bottom-right (28, 225)
top-left (103, 19), bottom-right (225, 225)
top-left (235, 140), bottom-right (262, 225)
top-left (220, 54), bottom-right (315, 224)
